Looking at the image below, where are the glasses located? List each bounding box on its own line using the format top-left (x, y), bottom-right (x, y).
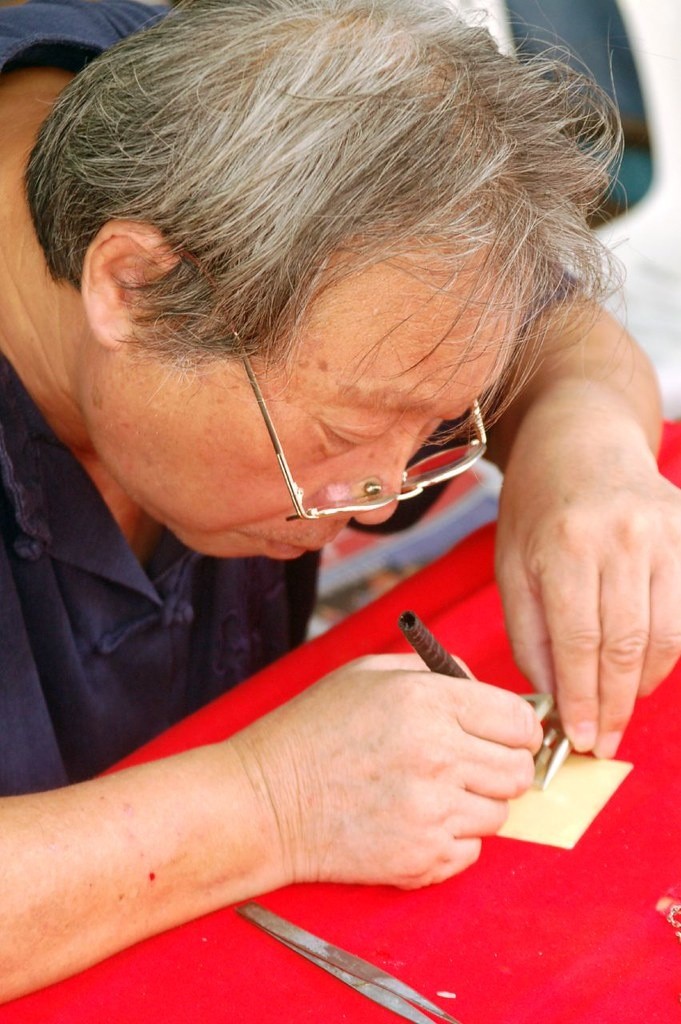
top-left (178, 248), bottom-right (487, 523)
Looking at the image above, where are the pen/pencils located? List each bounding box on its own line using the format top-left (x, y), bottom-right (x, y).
top-left (398, 610), bottom-right (470, 678)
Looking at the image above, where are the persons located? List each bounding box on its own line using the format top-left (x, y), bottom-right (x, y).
top-left (0, 0), bottom-right (681, 1004)
